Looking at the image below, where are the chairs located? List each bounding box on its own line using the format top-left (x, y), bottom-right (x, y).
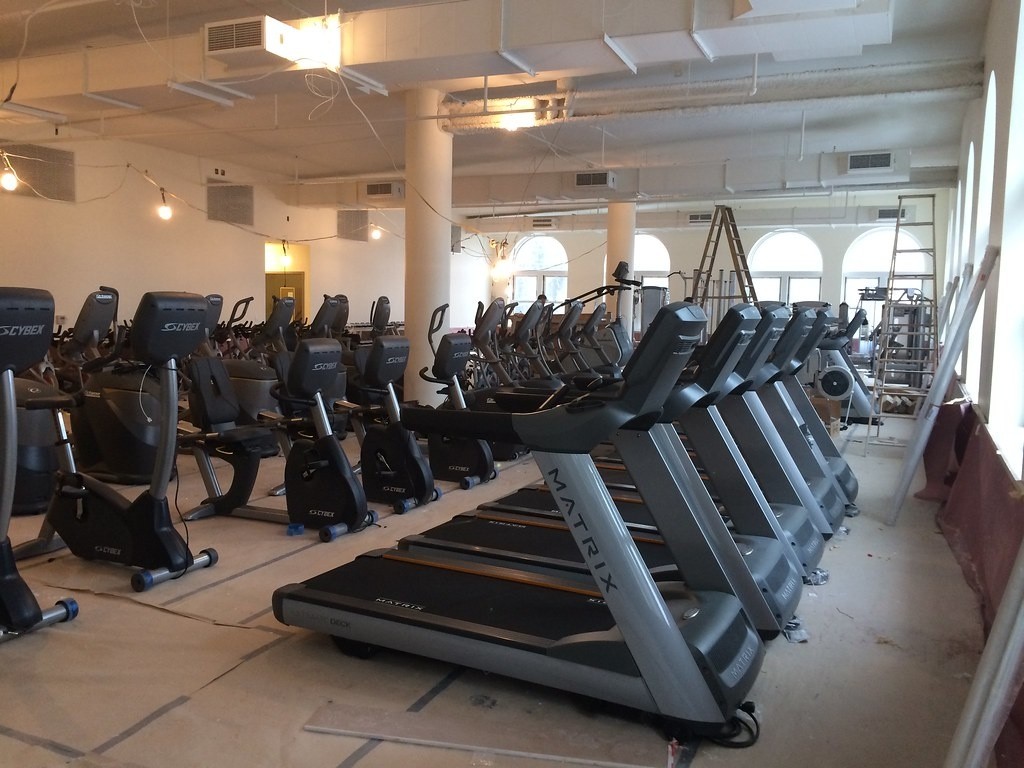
top-left (275, 352), bottom-right (347, 440)
top-left (0, 379), bottom-right (69, 515)
top-left (190, 356), bottom-right (280, 457)
top-left (337, 341), bottom-right (390, 408)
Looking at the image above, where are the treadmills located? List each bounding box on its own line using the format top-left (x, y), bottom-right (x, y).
top-left (271, 299), bottom-right (858, 736)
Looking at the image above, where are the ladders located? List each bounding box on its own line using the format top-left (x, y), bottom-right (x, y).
top-left (692, 205), bottom-right (763, 314)
top-left (864, 194), bottom-right (939, 458)
